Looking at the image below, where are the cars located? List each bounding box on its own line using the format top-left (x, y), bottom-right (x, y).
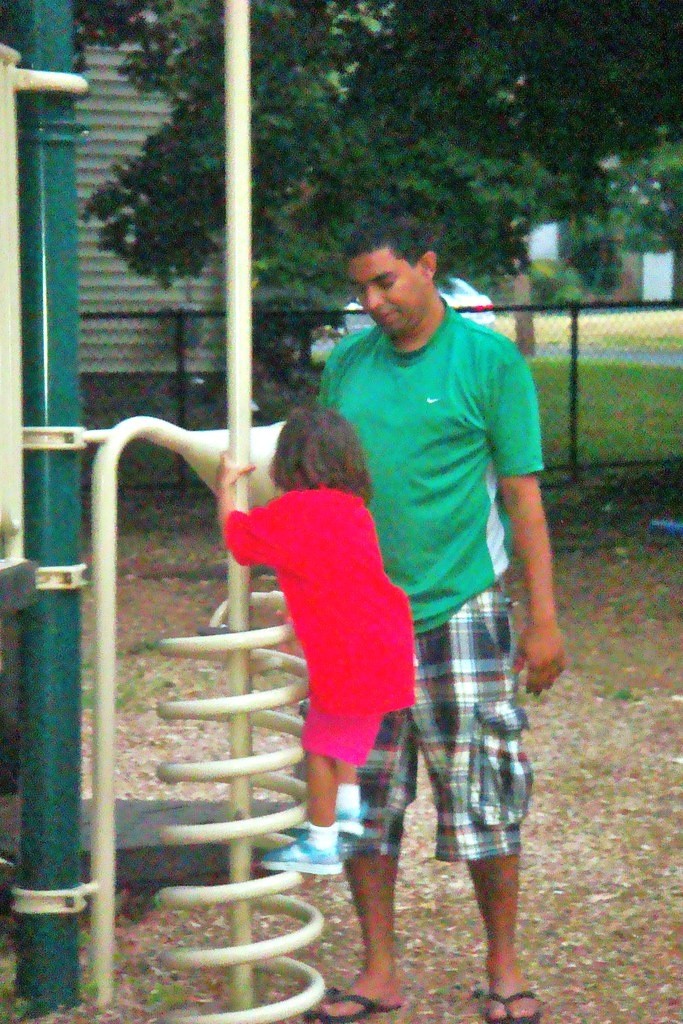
top-left (342, 276), bottom-right (498, 335)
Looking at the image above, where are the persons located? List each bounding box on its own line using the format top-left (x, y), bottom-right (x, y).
top-left (215, 406), bottom-right (415, 875)
top-left (316, 217), bottom-right (568, 1024)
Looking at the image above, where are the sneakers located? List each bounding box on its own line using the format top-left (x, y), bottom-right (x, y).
top-left (292, 807), bottom-right (363, 839)
top-left (261, 831), bottom-right (343, 876)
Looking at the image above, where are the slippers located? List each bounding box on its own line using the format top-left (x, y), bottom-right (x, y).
top-left (303, 985), bottom-right (402, 1024)
top-left (487, 993), bottom-right (540, 1023)
